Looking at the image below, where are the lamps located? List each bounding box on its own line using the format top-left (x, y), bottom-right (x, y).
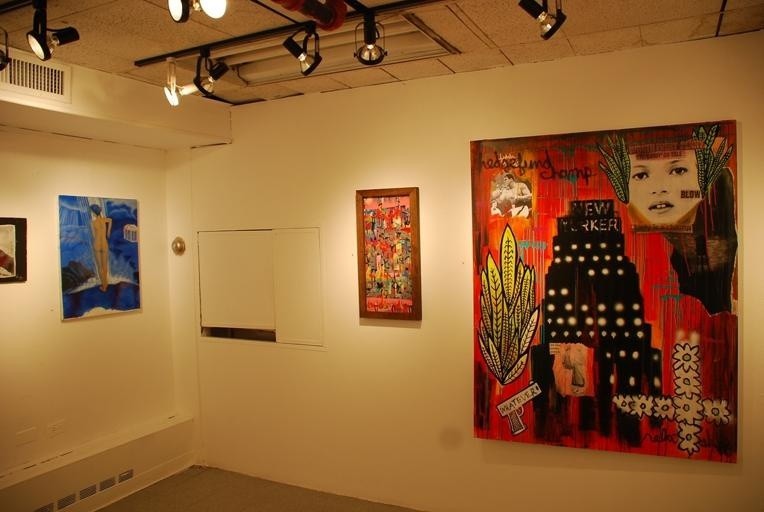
top-left (25, 1), bottom-right (568, 108)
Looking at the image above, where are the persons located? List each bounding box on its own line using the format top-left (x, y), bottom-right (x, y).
top-left (621, 137), bottom-right (706, 227)
top-left (363, 197), bottom-right (413, 298)
top-left (502, 175), bottom-right (531, 218)
top-left (88, 203), bottom-right (113, 293)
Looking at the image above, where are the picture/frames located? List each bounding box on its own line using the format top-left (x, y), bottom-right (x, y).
top-left (355, 187), bottom-right (422, 319)
